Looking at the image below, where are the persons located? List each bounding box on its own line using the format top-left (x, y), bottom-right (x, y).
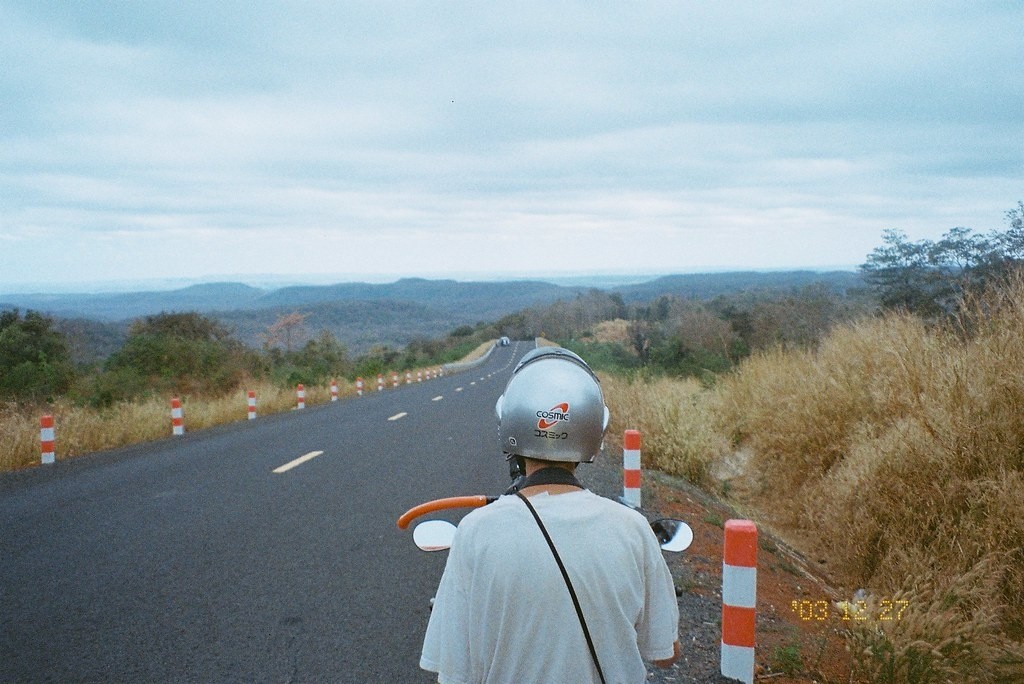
top-left (419, 347), bottom-right (681, 684)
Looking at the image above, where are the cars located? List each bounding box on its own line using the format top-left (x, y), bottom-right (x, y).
top-left (499, 337), bottom-right (510, 346)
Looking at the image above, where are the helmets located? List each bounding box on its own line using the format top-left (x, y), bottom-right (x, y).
top-left (655, 520), bottom-right (681, 543)
top-left (496, 351), bottom-right (609, 462)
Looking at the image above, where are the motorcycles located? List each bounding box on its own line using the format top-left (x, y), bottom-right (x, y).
top-left (398, 491), bottom-right (693, 617)
